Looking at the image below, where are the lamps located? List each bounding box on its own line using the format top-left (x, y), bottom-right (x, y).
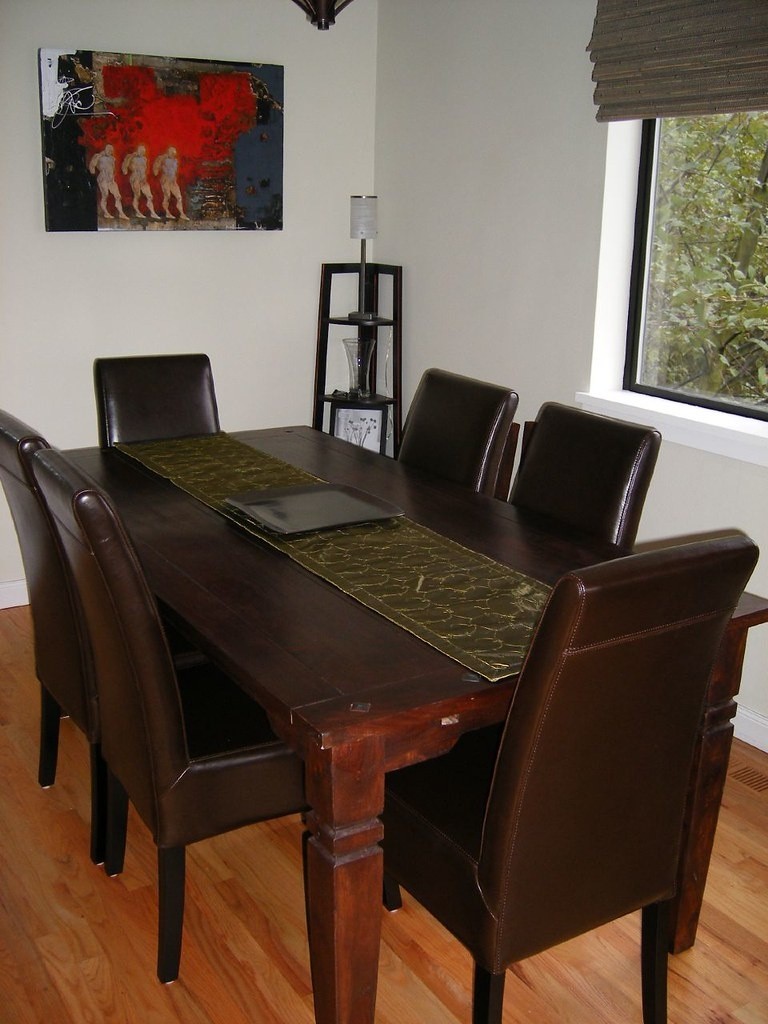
top-left (348, 195), bottom-right (378, 320)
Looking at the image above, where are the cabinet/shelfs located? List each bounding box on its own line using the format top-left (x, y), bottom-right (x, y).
top-left (312, 264), bottom-right (402, 461)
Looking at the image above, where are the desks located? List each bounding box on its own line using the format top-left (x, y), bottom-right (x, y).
top-left (60, 426), bottom-right (768, 1024)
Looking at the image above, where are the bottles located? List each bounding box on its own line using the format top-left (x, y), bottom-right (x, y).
top-left (343, 338), bottom-right (377, 397)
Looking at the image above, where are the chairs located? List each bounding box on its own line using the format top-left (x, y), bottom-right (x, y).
top-left (94, 354), bottom-right (221, 447)
top-left (507, 403), bottom-right (662, 550)
top-left (0, 409), bottom-right (206, 866)
top-left (32, 449), bottom-right (402, 984)
top-left (381, 529), bottom-right (759, 1023)
top-left (394, 368), bottom-right (520, 502)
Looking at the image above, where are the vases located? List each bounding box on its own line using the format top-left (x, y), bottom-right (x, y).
top-left (342, 338), bottom-right (376, 399)
